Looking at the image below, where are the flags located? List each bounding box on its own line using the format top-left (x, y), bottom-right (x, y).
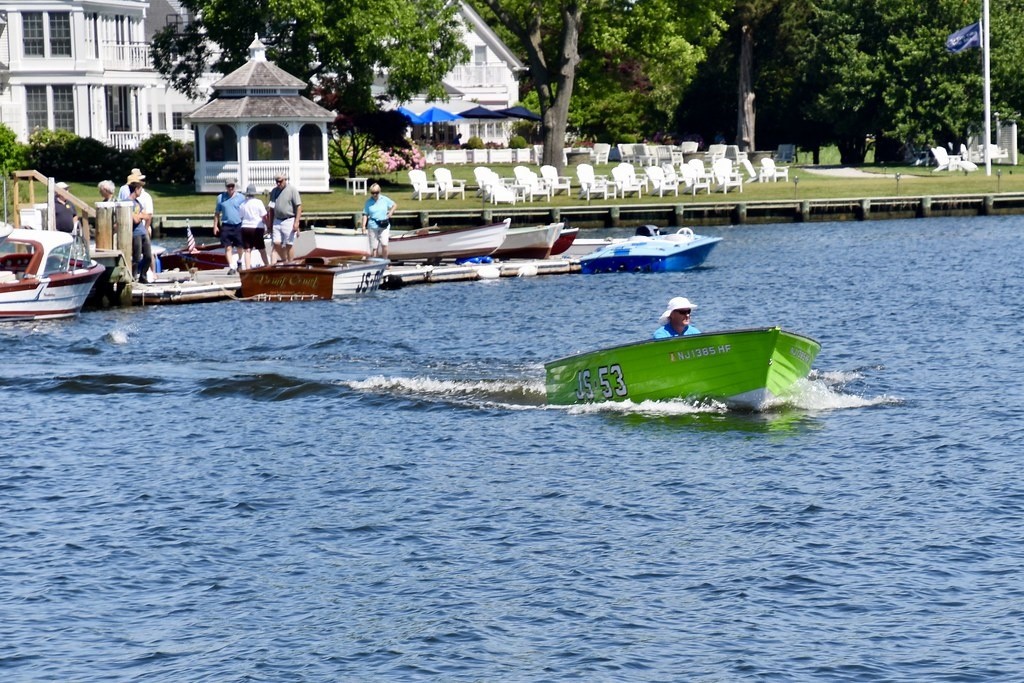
top-left (186, 226), bottom-right (196, 252)
top-left (944, 22), bottom-right (981, 55)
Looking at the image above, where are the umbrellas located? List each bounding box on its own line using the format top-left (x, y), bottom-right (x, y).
top-left (493, 105), bottom-right (543, 137)
top-left (457, 105), bottom-right (507, 138)
top-left (396, 106), bottom-right (424, 124)
top-left (419, 107), bottom-right (462, 147)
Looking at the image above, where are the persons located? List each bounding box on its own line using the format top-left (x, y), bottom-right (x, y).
top-left (361, 183), bottom-right (398, 270)
top-left (97, 180), bottom-right (119, 249)
top-left (125, 182), bottom-right (154, 286)
top-left (268, 172), bottom-right (303, 263)
top-left (214, 179), bottom-right (247, 275)
top-left (238, 183), bottom-right (271, 270)
top-left (53, 182), bottom-right (78, 233)
top-left (652, 296), bottom-right (700, 340)
top-left (453, 134), bottom-right (462, 144)
top-left (118, 168), bottom-right (157, 280)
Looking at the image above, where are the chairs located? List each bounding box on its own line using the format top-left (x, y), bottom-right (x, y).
top-left (409, 140), bottom-right (797, 205)
top-left (931, 144), bottom-right (984, 173)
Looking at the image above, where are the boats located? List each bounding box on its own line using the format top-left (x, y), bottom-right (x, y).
top-left (0, 180), bottom-right (581, 321)
top-left (544, 324), bottom-right (823, 414)
top-left (580, 224), bottom-right (722, 274)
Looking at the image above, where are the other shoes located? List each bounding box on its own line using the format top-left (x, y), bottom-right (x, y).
top-left (227, 268), bottom-right (236, 275)
top-left (237, 262), bottom-right (243, 272)
top-left (138, 278), bottom-right (149, 284)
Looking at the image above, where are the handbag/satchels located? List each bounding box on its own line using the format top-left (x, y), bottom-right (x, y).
top-left (370, 217), bottom-right (389, 227)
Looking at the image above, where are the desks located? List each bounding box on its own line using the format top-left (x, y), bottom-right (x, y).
top-left (344, 177), bottom-right (368, 197)
top-left (913, 151), bottom-right (933, 167)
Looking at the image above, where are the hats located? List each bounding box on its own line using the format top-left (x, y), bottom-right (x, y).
top-left (659, 296), bottom-right (697, 320)
top-left (126, 175), bottom-right (145, 186)
top-left (55, 182), bottom-right (69, 195)
top-left (225, 179), bottom-right (235, 186)
top-left (245, 185), bottom-right (256, 194)
top-left (131, 168), bottom-right (146, 180)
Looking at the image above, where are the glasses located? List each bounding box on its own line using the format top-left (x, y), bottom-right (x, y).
top-left (227, 185), bottom-right (234, 187)
top-left (371, 192), bottom-right (379, 194)
top-left (276, 178), bottom-right (284, 183)
top-left (673, 309), bottom-right (691, 315)
